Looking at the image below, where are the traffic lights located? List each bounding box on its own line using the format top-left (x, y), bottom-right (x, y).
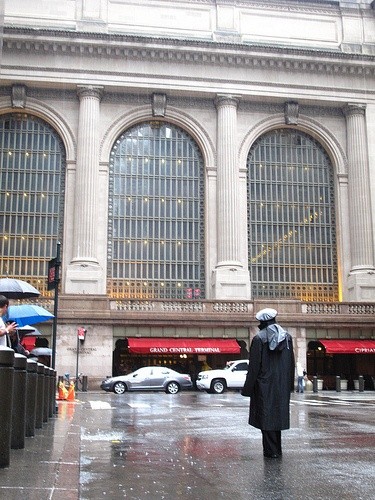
top-left (46, 258), bottom-right (58, 291)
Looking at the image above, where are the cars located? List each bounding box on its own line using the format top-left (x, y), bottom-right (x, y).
top-left (100, 366), bottom-right (193, 395)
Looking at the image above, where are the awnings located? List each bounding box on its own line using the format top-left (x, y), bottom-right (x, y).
top-left (317, 339), bottom-right (375, 354)
top-left (126, 338), bottom-right (241, 355)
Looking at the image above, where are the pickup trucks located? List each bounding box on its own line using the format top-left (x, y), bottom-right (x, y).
top-left (195, 359), bottom-right (251, 395)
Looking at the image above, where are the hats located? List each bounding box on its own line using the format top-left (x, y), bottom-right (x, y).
top-left (256, 308), bottom-right (278, 320)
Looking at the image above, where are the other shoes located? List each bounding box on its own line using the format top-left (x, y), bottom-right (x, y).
top-left (296, 391), bottom-right (299, 392)
top-left (265, 452), bottom-right (282, 458)
top-left (300, 392), bottom-right (303, 393)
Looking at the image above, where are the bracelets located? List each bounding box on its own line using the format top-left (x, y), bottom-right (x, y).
top-left (6, 329), bottom-right (9, 334)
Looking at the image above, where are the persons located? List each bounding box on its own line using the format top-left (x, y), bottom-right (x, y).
top-left (240, 307), bottom-right (296, 459)
top-left (0, 296), bottom-right (31, 357)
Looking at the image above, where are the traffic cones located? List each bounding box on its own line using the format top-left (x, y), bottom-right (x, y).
top-left (55, 379), bottom-right (68, 401)
top-left (65, 379), bottom-right (78, 403)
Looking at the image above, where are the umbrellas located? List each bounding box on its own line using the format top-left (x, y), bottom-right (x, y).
top-left (0, 276), bottom-right (42, 295)
top-left (31, 347), bottom-right (52, 356)
top-left (2, 304), bottom-right (56, 337)
